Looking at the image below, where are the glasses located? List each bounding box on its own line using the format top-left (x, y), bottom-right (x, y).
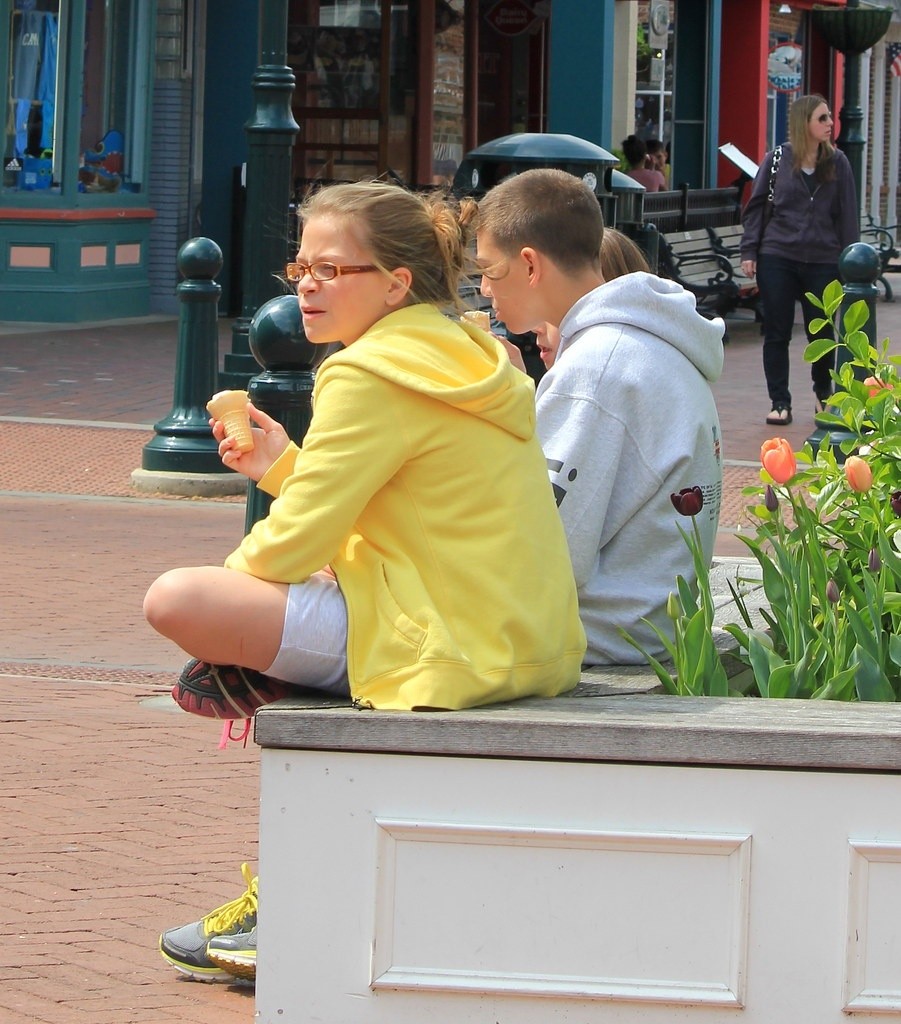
top-left (809, 111), bottom-right (832, 125)
top-left (285, 261), bottom-right (415, 289)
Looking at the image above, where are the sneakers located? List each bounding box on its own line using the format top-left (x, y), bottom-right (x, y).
top-left (158, 863), bottom-right (257, 984)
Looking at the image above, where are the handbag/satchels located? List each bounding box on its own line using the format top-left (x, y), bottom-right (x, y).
top-left (759, 146), bottom-right (782, 237)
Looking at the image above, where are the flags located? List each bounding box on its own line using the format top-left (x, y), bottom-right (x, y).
top-left (890, 42), bottom-right (901, 76)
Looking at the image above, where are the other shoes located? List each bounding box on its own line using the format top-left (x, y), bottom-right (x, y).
top-left (171, 658), bottom-right (329, 750)
top-left (766, 403), bottom-right (792, 425)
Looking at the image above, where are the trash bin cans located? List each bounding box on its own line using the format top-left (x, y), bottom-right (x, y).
top-left (611, 170), bottom-right (660, 272)
top-left (467, 132), bottom-right (620, 229)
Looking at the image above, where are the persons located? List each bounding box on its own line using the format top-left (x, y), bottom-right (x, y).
top-left (532, 227), bottom-right (651, 369)
top-left (143, 179), bottom-right (587, 720)
top-left (161, 168), bottom-right (726, 985)
top-left (622, 135), bottom-right (669, 193)
top-left (738, 96), bottom-right (859, 425)
top-left (646, 140), bottom-right (671, 193)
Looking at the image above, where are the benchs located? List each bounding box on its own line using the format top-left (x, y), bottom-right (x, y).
top-left (860, 215), bottom-right (901, 300)
top-left (641, 223), bottom-right (765, 344)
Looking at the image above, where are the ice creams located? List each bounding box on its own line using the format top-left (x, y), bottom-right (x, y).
top-left (460, 310), bottom-right (491, 332)
top-left (206, 389), bottom-right (254, 453)
